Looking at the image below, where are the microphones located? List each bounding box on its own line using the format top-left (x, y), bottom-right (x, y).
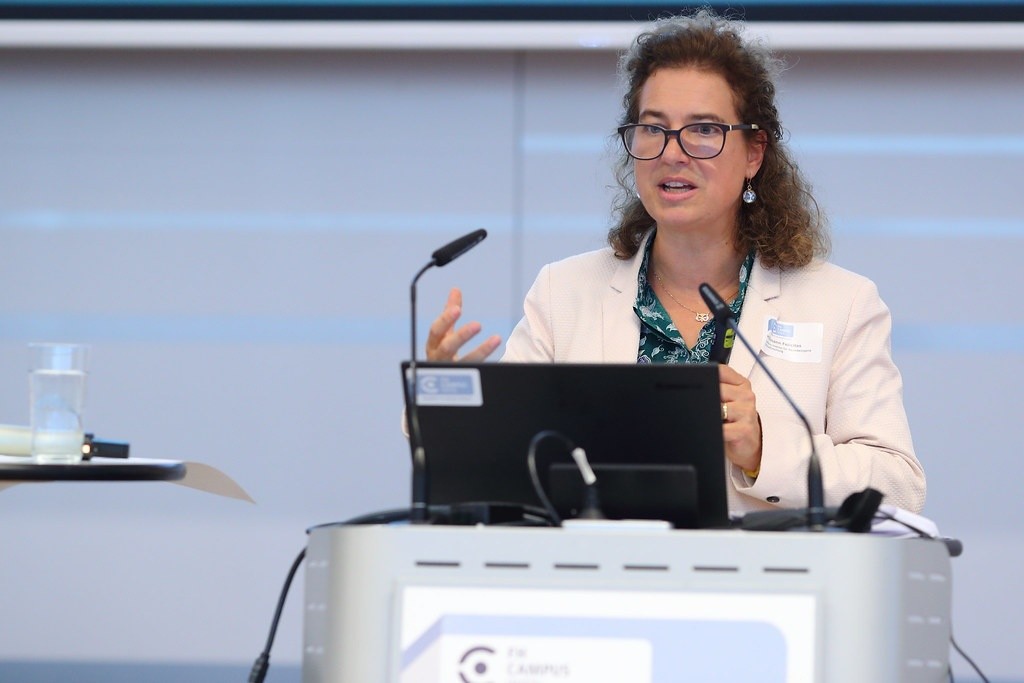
top-left (699, 281), bottom-right (843, 532)
top-left (406, 229), bottom-right (488, 525)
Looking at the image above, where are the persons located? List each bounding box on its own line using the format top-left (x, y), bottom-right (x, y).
top-left (426, 11), bottom-right (928, 516)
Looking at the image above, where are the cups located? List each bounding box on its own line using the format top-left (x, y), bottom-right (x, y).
top-left (24, 341), bottom-right (90, 461)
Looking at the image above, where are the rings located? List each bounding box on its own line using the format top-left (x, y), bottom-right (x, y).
top-left (723, 403), bottom-right (727, 419)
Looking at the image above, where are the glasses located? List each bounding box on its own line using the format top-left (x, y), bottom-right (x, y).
top-left (618, 123), bottom-right (759, 160)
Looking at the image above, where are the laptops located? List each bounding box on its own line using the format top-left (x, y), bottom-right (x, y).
top-left (401, 362), bottom-right (740, 534)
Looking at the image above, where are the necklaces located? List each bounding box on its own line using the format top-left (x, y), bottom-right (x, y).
top-left (653, 275), bottom-right (739, 323)
